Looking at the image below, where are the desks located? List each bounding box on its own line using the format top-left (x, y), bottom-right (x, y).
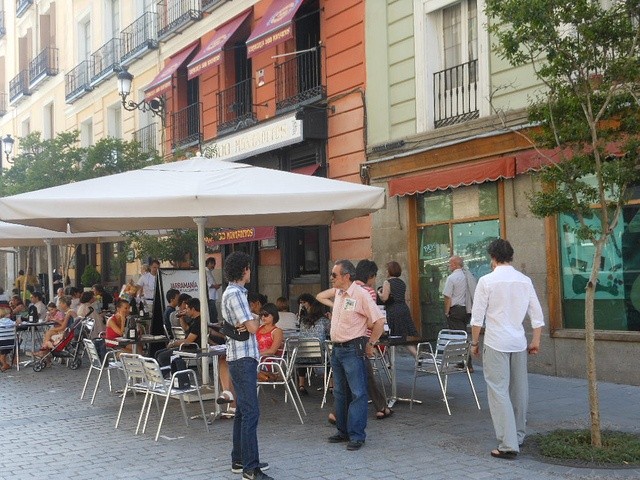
top-left (118, 333), bottom-right (168, 401)
top-left (172, 344), bottom-right (233, 424)
top-left (371, 334), bottom-right (424, 409)
top-left (14, 318), bottom-right (59, 370)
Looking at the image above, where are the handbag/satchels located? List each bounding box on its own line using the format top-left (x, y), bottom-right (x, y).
top-left (258, 369), bottom-right (278, 382)
top-left (377, 286), bottom-right (394, 305)
top-left (172, 357), bottom-right (190, 389)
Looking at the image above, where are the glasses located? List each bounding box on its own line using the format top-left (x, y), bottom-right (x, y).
top-left (209, 260), bottom-right (216, 264)
top-left (331, 271), bottom-right (342, 280)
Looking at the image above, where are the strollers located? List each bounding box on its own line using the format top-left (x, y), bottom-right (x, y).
top-left (32, 305), bottom-right (95, 372)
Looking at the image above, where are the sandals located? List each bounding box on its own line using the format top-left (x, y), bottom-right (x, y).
top-left (328, 410), bottom-right (337, 425)
top-left (299, 385), bottom-right (307, 395)
top-left (328, 386), bottom-right (334, 393)
top-left (218, 390), bottom-right (234, 404)
top-left (223, 407), bottom-right (236, 419)
top-left (377, 405), bottom-right (395, 419)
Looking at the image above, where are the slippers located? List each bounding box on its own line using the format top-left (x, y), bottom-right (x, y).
top-left (491, 447), bottom-right (518, 457)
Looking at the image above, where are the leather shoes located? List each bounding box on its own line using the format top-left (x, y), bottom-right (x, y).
top-left (346, 440), bottom-right (365, 450)
top-left (328, 433), bottom-right (349, 443)
top-left (454, 363), bottom-right (463, 366)
top-left (464, 368), bottom-right (474, 373)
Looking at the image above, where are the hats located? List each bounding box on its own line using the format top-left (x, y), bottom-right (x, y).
top-left (259, 311), bottom-right (272, 317)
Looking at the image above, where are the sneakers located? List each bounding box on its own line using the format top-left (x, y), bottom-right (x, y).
top-left (242, 468), bottom-right (274, 480)
top-left (232, 460), bottom-right (269, 472)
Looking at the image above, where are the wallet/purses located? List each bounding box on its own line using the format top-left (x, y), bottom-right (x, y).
top-left (219, 319), bottom-right (249, 341)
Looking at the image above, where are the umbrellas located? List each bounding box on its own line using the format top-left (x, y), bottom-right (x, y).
top-left (0, 153), bottom-right (388, 385)
top-left (0, 219), bottom-right (182, 304)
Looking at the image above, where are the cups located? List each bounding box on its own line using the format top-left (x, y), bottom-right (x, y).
top-left (16, 314), bottom-right (21, 323)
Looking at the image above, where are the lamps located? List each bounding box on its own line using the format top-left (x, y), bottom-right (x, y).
top-left (114, 65), bottom-right (166, 119)
top-left (1, 133), bottom-right (34, 164)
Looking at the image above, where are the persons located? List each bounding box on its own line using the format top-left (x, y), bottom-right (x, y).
top-left (442, 255), bottom-right (474, 373)
top-left (92, 283), bottom-right (113, 311)
top-left (44, 302), bottom-right (64, 367)
top-left (114, 278), bottom-right (138, 304)
top-left (154, 298), bottom-right (202, 378)
top-left (23, 267), bottom-right (39, 294)
top-left (163, 289), bottom-right (180, 338)
top-left (9, 295), bottom-right (29, 324)
top-left (470, 239), bottom-right (545, 458)
top-left (205, 256), bottom-right (223, 325)
top-left (54, 288), bottom-right (68, 303)
top-left (69, 287), bottom-right (82, 309)
top-left (81, 292), bottom-right (103, 313)
top-left (217, 303), bottom-right (282, 419)
top-left (137, 260), bottom-right (159, 342)
top-left (170, 294), bottom-right (225, 346)
top-left (220, 252), bottom-right (274, 480)
top-left (16, 291), bottom-right (46, 331)
top-left (376, 262), bottom-right (423, 367)
top-left (295, 293), bottom-right (332, 394)
top-left (104, 298), bottom-right (134, 365)
top-left (248, 291), bottom-right (269, 316)
top-left (32, 298), bottom-right (78, 357)
top-left (0, 305), bottom-right (16, 371)
top-left (315, 260), bottom-right (396, 423)
top-left (328, 259), bottom-right (383, 450)
top-left (15, 270), bottom-right (26, 291)
top-left (276, 296), bottom-right (290, 312)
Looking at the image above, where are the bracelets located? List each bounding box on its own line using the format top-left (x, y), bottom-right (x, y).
top-left (369, 339), bottom-right (376, 346)
top-left (471, 341), bottom-right (479, 346)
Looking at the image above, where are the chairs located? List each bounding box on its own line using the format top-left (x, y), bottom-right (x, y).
top-left (409, 339), bottom-right (482, 416)
top-left (80, 338), bottom-right (139, 405)
top-left (77, 315), bottom-right (96, 366)
top-left (418, 328), bottom-right (469, 400)
top-left (98, 330), bottom-right (127, 392)
top-left (163, 324), bottom-right (170, 339)
top-left (256, 338), bottom-right (290, 399)
top-left (372, 304), bottom-right (393, 386)
top-left (0, 322), bottom-right (20, 371)
top-left (255, 347), bottom-right (308, 425)
top-left (114, 352), bottom-right (190, 430)
top-left (286, 336), bottom-right (330, 404)
top-left (137, 355), bottom-right (211, 442)
top-left (179, 331), bottom-right (212, 352)
top-left (171, 326), bottom-right (186, 340)
top-left (319, 339), bottom-right (388, 410)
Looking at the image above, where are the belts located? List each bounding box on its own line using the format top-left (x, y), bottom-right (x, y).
top-left (450, 304), bottom-right (467, 308)
top-left (332, 336), bottom-right (363, 348)
top-left (146, 298), bottom-right (154, 300)
